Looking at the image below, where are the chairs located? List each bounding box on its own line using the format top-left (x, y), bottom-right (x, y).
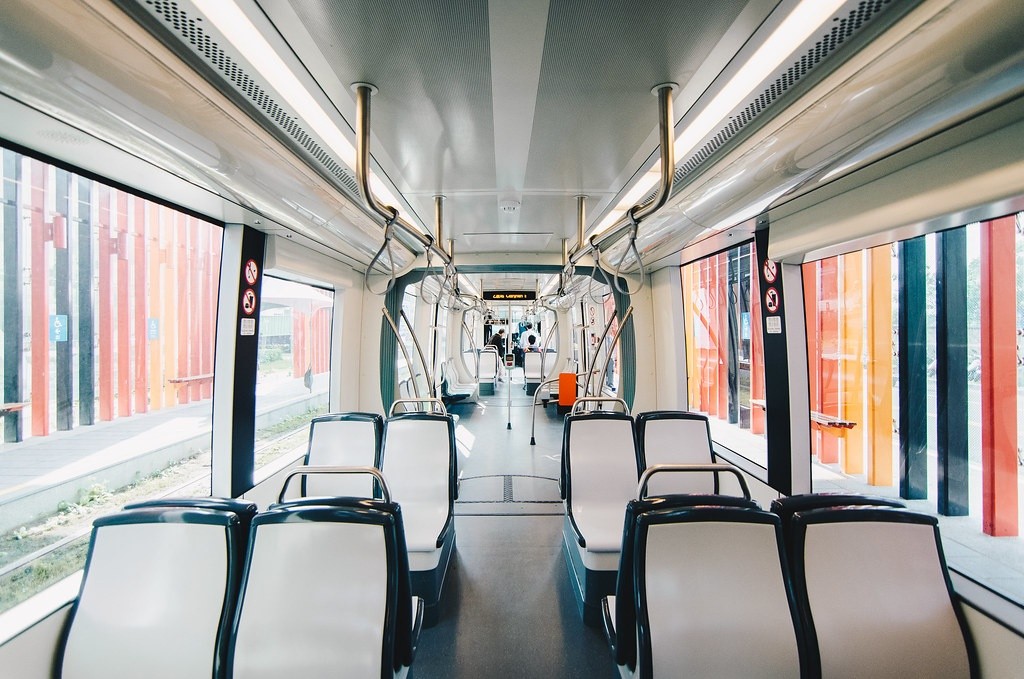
top-left (787, 504), bottom-right (976, 679)
top-left (635, 410), bottom-right (719, 495)
top-left (633, 504), bottom-right (808, 679)
top-left (558, 410), bottom-right (627, 501)
top-left (599, 495), bottom-right (763, 667)
top-left (301, 412), bottom-right (383, 498)
top-left (267, 496), bottom-right (424, 679)
top-left (121, 494), bottom-right (259, 582)
top-left (565, 414), bottom-right (644, 552)
top-left (400, 348), bottom-right (579, 422)
top-left (53, 507), bottom-right (244, 679)
top-left (224, 505), bottom-right (399, 679)
top-left (377, 412), bottom-right (456, 554)
top-left (770, 491), bottom-right (907, 560)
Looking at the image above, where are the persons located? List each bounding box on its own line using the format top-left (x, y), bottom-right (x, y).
top-left (484, 328), bottom-right (505, 366)
top-left (518, 322), bottom-right (543, 390)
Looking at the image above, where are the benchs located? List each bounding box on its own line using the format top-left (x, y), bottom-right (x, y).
top-left (749, 399), bottom-right (857, 430)
top-left (0, 402), bottom-right (31, 443)
top-left (168, 373), bottom-right (214, 406)
